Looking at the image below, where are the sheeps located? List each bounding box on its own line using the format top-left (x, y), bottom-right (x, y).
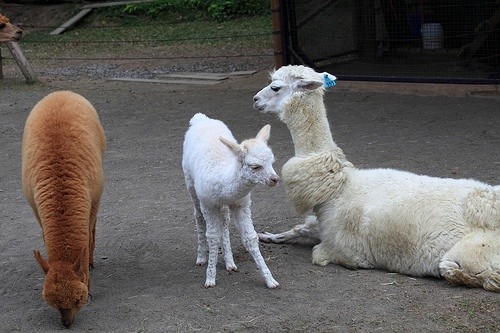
top-left (180, 111), bottom-right (280, 289)
top-left (21, 91), bottom-right (108, 328)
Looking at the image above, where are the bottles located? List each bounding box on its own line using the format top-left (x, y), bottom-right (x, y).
top-left (377, 42), bottom-right (383, 57)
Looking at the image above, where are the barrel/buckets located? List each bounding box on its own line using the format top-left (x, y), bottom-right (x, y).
top-left (421, 23), bottom-right (446, 49)
